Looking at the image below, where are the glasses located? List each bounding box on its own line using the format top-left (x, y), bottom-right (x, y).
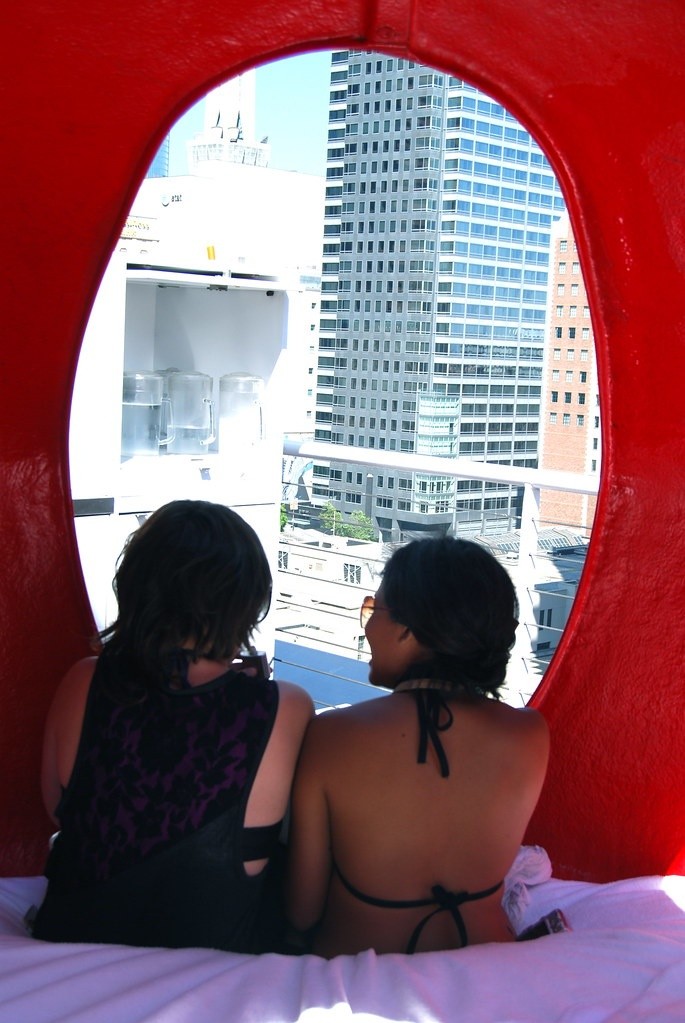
top-left (360, 596), bottom-right (390, 628)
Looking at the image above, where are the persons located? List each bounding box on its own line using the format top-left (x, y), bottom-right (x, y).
top-left (32, 499), bottom-right (316, 956)
top-left (287, 538), bottom-right (552, 960)
